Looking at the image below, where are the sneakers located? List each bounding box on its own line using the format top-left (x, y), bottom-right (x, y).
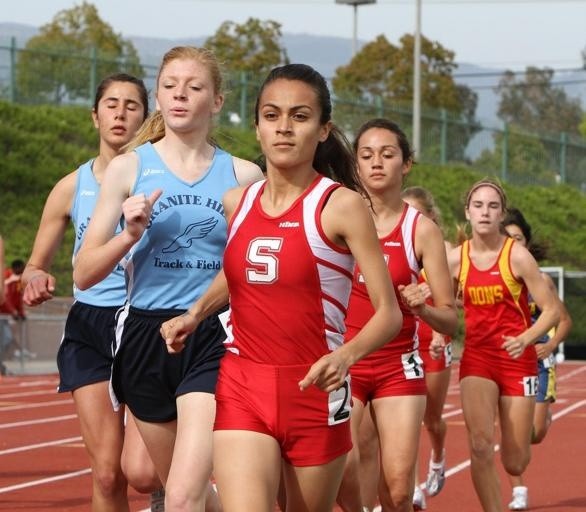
top-left (427, 452), bottom-right (445, 496)
top-left (509, 487), bottom-right (527, 510)
top-left (414, 489), bottom-right (426, 509)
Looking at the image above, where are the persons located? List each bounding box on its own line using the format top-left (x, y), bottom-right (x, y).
top-left (1, 259), bottom-right (24, 375)
top-left (0, 236), bottom-right (6, 307)
top-left (350, 119), bottom-right (571, 512)
top-left (73, 45), bottom-right (266, 512)
top-left (20, 75), bottom-right (165, 512)
top-left (156, 64), bottom-right (404, 512)
top-left (13, 293), bottom-right (36, 358)
